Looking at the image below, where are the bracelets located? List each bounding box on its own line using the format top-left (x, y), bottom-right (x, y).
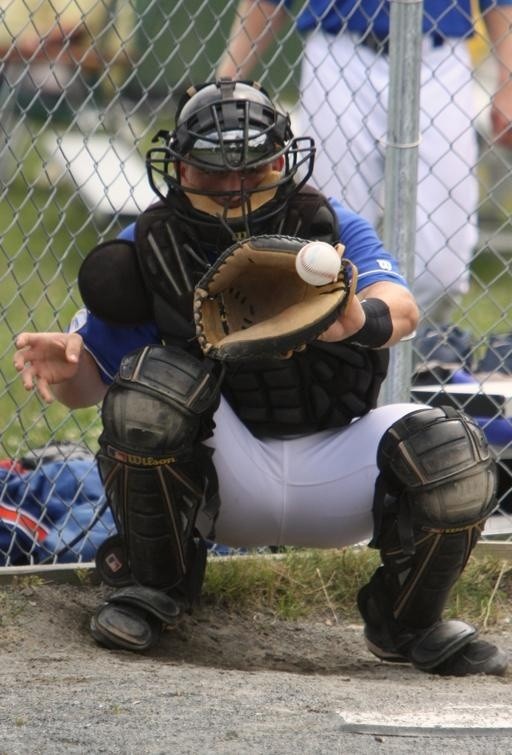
top-left (348, 296), bottom-right (393, 349)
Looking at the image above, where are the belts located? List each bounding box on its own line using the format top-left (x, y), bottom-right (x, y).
top-left (315, 22), bottom-right (445, 56)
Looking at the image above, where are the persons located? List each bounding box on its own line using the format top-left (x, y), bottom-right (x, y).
top-left (208, 0), bottom-right (512, 333)
top-left (13, 75), bottom-right (506, 678)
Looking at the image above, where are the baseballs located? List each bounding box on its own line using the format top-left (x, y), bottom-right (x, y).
top-left (294, 241), bottom-right (341, 287)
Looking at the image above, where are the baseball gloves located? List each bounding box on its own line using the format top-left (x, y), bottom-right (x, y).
top-left (194, 233), bottom-right (358, 362)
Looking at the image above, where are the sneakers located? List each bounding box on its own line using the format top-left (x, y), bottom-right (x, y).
top-left (410, 323), bottom-right (512, 385)
top-left (89, 587), bottom-right (178, 653)
top-left (361, 613), bottom-right (509, 682)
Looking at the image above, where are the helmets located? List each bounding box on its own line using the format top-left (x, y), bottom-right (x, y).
top-left (172, 79), bottom-right (291, 239)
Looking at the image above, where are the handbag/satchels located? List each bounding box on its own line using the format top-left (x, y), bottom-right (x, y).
top-left (0, 439), bottom-right (290, 567)
